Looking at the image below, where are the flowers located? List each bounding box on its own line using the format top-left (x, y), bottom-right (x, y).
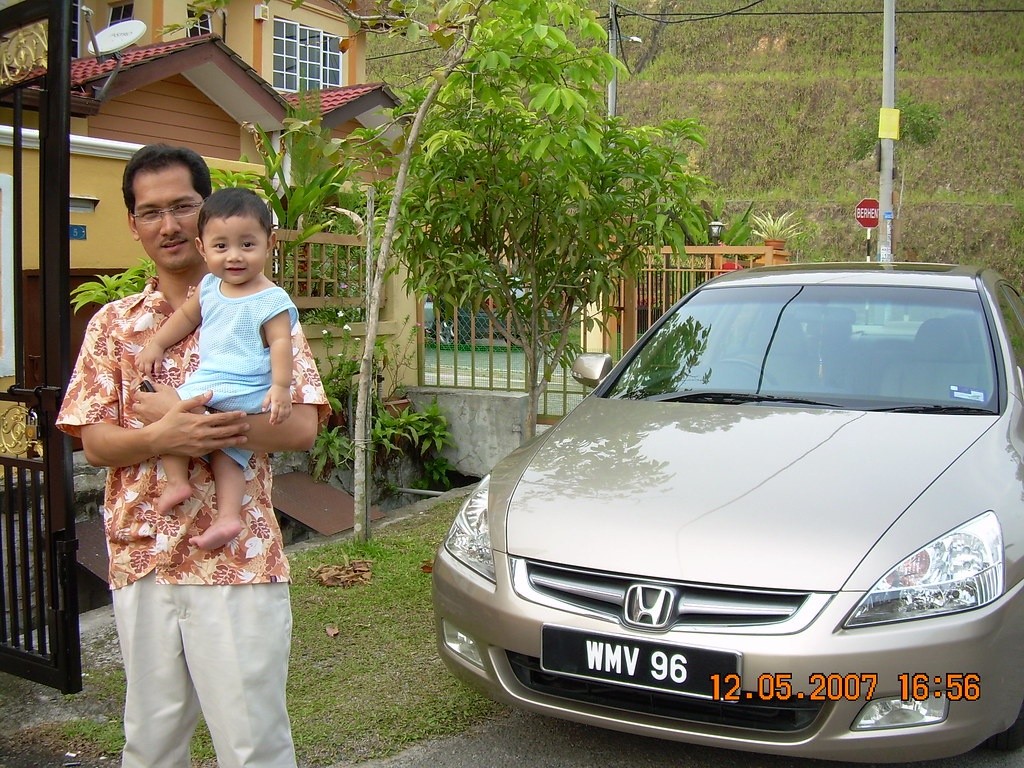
top-left (314, 324), bottom-right (362, 414)
top-left (372, 313), bottom-right (431, 401)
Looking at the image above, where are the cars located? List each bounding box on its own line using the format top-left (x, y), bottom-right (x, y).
top-left (430, 266), bottom-right (1024, 765)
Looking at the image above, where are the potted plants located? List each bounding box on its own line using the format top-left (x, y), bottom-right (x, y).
top-left (750, 211), bottom-right (803, 250)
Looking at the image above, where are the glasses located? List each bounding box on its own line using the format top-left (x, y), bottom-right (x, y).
top-left (131, 201), bottom-right (202, 224)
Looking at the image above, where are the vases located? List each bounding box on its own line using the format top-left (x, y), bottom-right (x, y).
top-left (381, 398), bottom-right (411, 418)
top-left (328, 412), bottom-right (346, 433)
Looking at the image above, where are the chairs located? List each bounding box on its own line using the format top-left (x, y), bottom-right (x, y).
top-left (881, 318), bottom-right (982, 404)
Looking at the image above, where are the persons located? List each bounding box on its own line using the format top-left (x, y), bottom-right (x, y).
top-left (53, 141), bottom-right (335, 768)
top-left (136, 188), bottom-right (300, 549)
